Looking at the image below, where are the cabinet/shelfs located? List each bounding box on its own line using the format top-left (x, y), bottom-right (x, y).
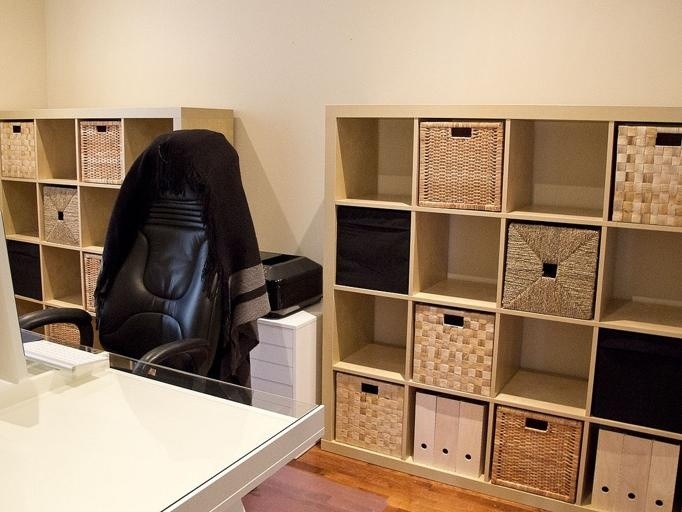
top-left (250, 311), bottom-right (323, 416)
top-left (320, 106), bottom-right (682, 512)
top-left (0, 107), bottom-right (234, 369)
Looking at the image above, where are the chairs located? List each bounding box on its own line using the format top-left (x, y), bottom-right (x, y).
top-left (18, 129), bottom-right (239, 392)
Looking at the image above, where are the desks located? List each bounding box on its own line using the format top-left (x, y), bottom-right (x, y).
top-left (0, 328), bottom-right (326, 512)
